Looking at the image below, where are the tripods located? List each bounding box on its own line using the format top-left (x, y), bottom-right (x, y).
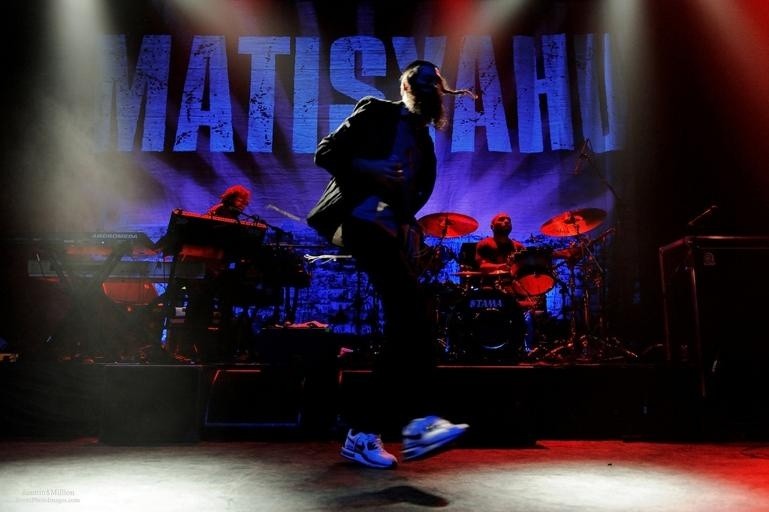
top-left (526, 265), bottom-right (640, 366)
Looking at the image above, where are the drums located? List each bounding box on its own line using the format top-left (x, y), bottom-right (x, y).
top-left (448, 287), bottom-right (528, 362)
top-left (512, 245), bottom-right (556, 296)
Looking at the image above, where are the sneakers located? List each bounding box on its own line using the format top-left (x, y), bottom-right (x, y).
top-left (340, 429), bottom-right (398, 469)
top-left (400, 416), bottom-right (470, 461)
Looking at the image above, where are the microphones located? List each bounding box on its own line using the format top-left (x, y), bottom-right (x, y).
top-left (687, 205), bottom-right (719, 227)
top-left (573, 139), bottom-right (590, 177)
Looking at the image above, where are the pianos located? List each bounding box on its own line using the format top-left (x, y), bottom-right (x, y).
top-left (33, 230), bottom-right (159, 255)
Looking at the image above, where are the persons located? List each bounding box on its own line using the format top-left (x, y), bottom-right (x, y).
top-left (476, 213), bottom-right (571, 272)
top-left (307, 61), bottom-right (478, 469)
top-left (207, 185), bottom-right (252, 220)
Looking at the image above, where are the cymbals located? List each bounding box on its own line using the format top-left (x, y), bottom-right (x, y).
top-left (552, 247), bottom-right (579, 257)
top-left (417, 211), bottom-right (480, 239)
top-left (451, 272), bottom-right (482, 276)
top-left (538, 208), bottom-right (605, 237)
